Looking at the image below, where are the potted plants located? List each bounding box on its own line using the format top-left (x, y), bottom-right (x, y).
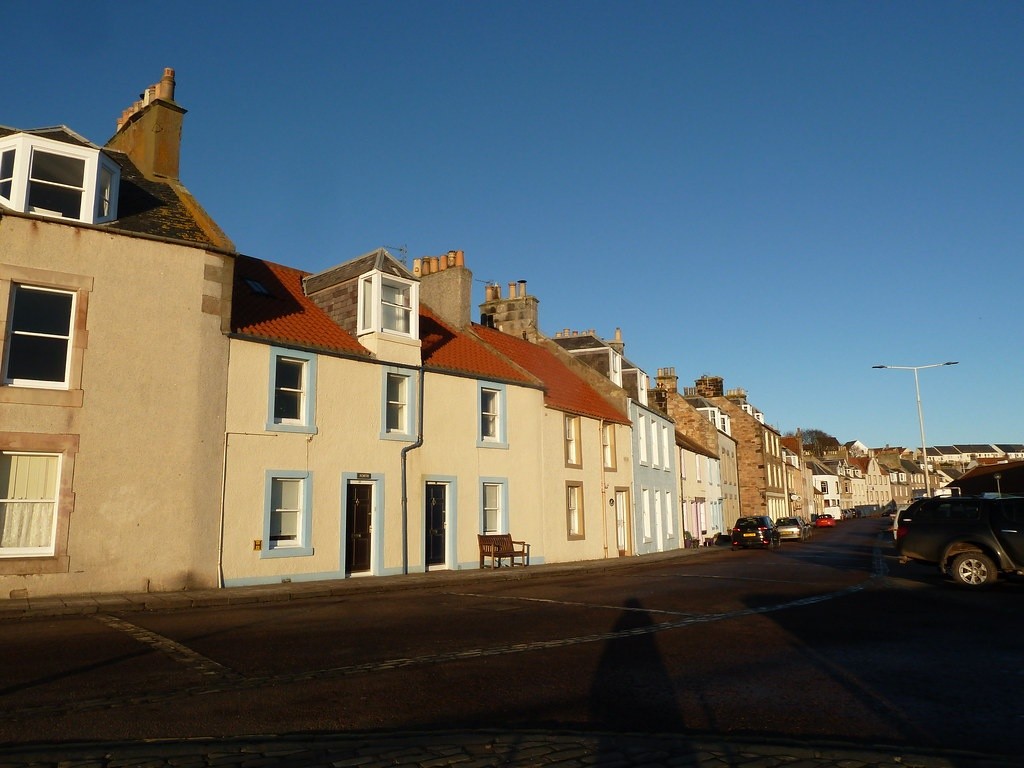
top-left (684, 531), bottom-right (693, 549)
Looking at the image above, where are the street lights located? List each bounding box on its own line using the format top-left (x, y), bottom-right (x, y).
top-left (872, 362), bottom-right (959, 498)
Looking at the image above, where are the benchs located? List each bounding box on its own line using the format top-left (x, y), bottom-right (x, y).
top-left (477, 533), bottom-right (526, 571)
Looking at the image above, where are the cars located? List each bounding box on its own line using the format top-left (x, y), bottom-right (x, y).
top-left (775, 515), bottom-right (812, 542)
top-left (892, 473), bottom-right (1024, 587)
top-left (842, 509), bottom-right (858, 519)
top-left (816, 514), bottom-right (836, 527)
top-left (730, 515), bottom-right (780, 550)
